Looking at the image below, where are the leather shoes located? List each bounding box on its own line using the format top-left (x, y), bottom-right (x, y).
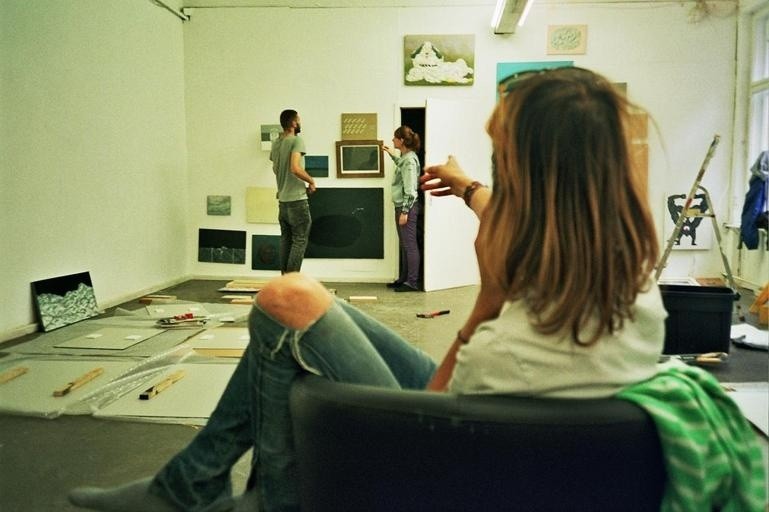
top-left (386, 282), bottom-right (403, 288)
top-left (394, 284), bottom-right (416, 291)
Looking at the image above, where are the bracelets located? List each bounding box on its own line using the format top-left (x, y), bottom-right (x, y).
top-left (463, 179), bottom-right (487, 208)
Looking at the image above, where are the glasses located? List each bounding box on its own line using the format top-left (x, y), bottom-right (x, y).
top-left (497, 66), bottom-right (599, 127)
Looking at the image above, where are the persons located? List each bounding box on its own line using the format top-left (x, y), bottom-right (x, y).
top-left (64, 63), bottom-right (671, 510)
top-left (268, 110), bottom-right (317, 276)
top-left (381, 125), bottom-right (422, 293)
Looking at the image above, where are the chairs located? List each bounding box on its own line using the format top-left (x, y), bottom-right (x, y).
top-left (286, 372), bottom-right (666, 508)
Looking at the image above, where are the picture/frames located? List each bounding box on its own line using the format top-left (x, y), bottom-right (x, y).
top-left (335, 140), bottom-right (384, 179)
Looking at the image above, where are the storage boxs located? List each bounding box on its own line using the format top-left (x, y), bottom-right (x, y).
top-left (658, 284), bottom-right (741, 354)
